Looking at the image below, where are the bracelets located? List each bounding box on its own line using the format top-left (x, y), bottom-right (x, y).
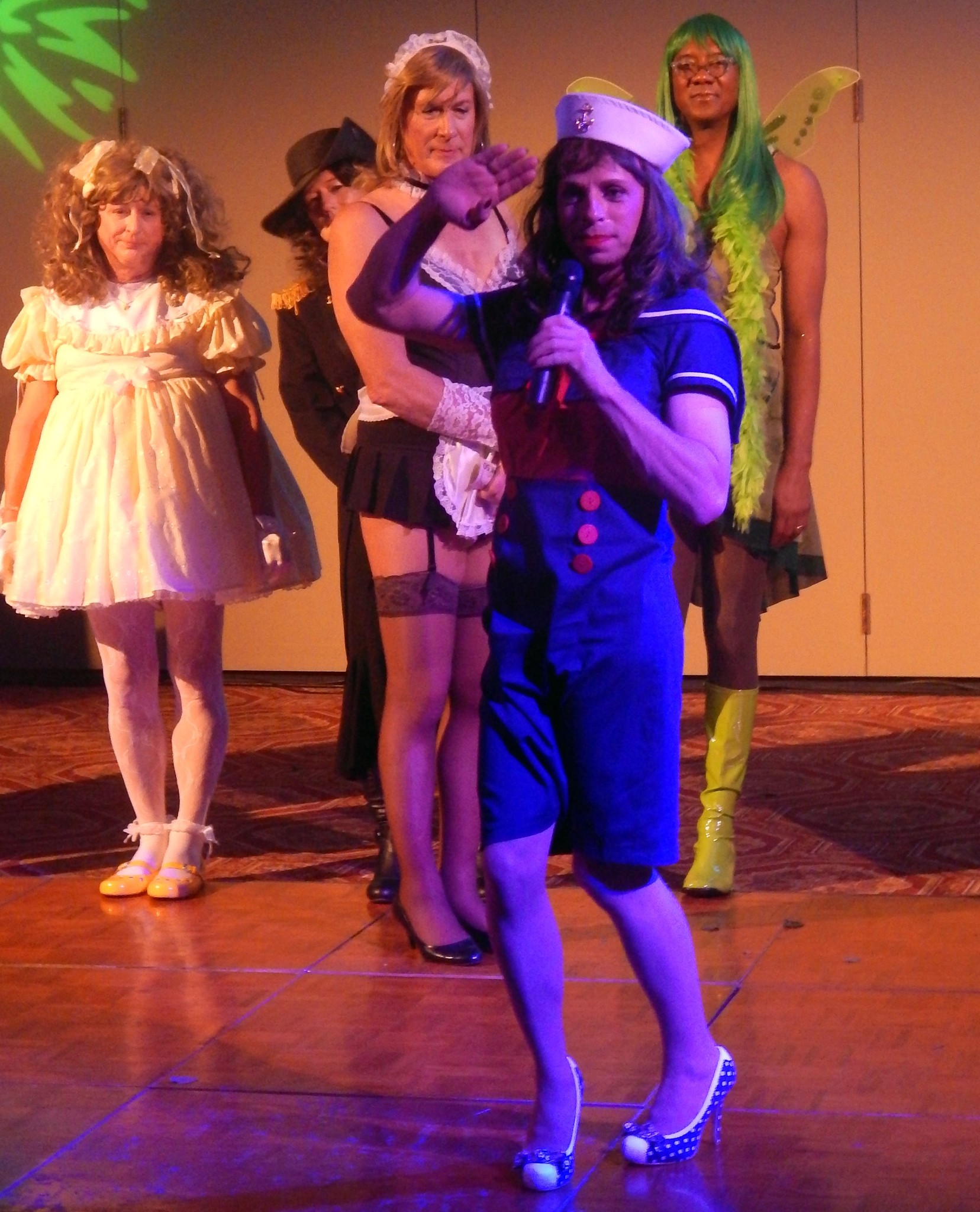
top-left (3, 505), bottom-right (20, 512)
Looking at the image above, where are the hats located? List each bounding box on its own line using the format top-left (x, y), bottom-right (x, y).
top-left (384, 29), bottom-right (494, 109)
top-left (262, 118), bottom-right (377, 239)
top-left (554, 92), bottom-right (691, 174)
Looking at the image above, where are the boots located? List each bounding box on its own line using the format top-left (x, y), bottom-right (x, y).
top-left (356, 772), bottom-right (404, 903)
top-left (682, 680), bottom-right (760, 897)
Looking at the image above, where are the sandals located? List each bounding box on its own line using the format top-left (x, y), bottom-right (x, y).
top-left (98, 837), bottom-right (170, 895)
top-left (149, 839), bottom-right (215, 899)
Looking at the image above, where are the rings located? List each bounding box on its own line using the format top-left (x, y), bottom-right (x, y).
top-left (798, 521), bottom-right (804, 530)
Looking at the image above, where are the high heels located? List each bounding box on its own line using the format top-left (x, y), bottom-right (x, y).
top-left (392, 895), bottom-right (481, 966)
top-left (460, 910), bottom-right (495, 953)
top-left (512, 1056), bottom-right (583, 1192)
top-left (621, 1046), bottom-right (736, 1165)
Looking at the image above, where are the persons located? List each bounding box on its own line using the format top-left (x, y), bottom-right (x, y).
top-left (347, 94), bottom-right (737, 1190)
top-left (0, 139), bottom-right (322, 899)
top-left (660, 13), bottom-right (827, 891)
top-left (326, 28), bottom-right (531, 967)
top-left (261, 117), bottom-right (399, 902)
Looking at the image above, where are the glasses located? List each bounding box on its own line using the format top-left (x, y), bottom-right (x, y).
top-left (670, 56), bottom-right (736, 79)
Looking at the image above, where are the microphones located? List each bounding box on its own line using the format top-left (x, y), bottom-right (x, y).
top-left (527, 259), bottom-right (586, 411)
